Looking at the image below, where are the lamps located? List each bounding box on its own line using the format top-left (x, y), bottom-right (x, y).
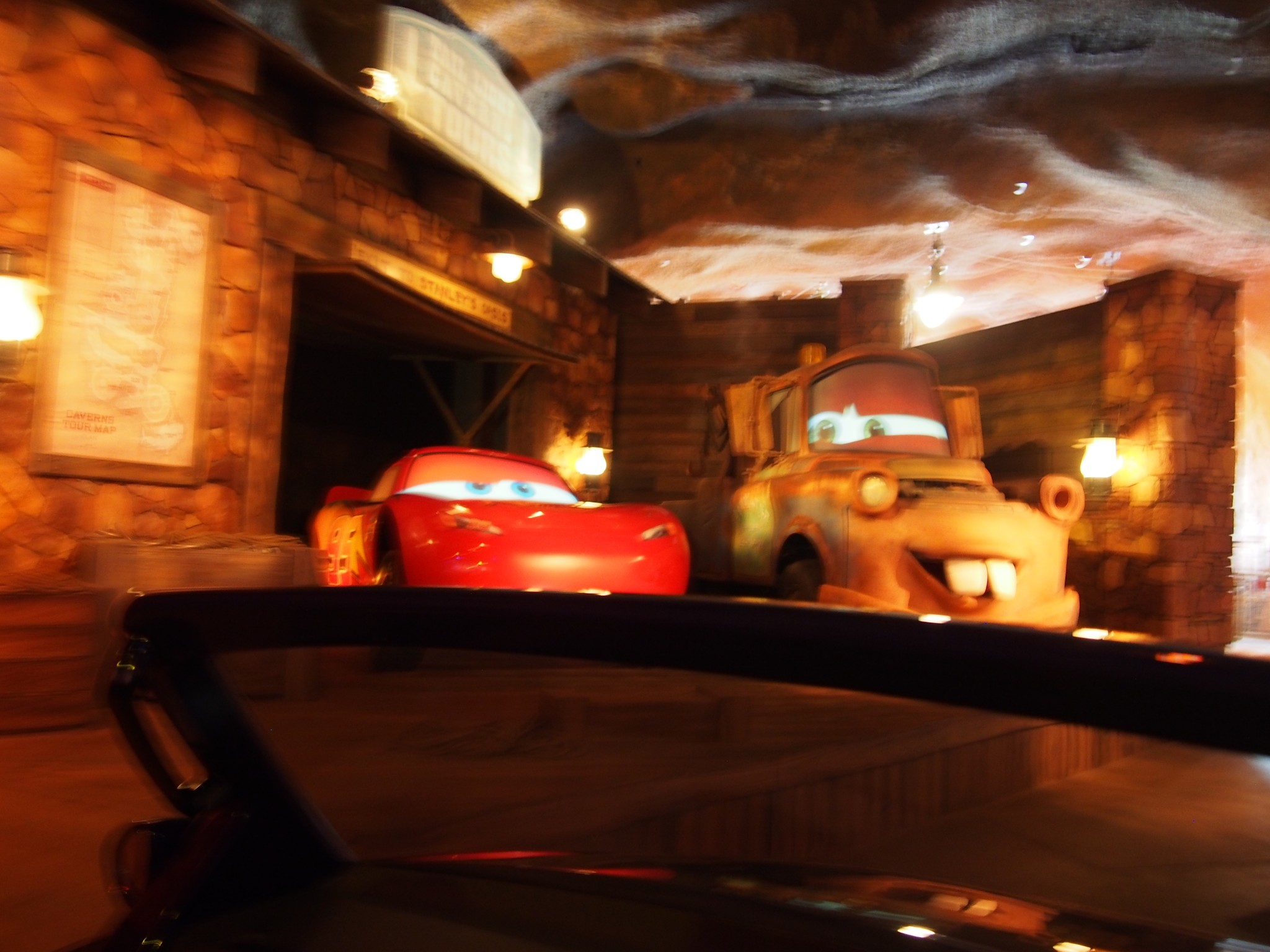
top-left (463, 221), bottom-right (534, 285)
top-left (0, 243), bottom-right (51, 344)
top-left (574, 430), bottom-right (615, 477)
top-left (343, 65), bottom-right (402, 104)
top-left (1070, 416), bottom-right (1123, 480)
